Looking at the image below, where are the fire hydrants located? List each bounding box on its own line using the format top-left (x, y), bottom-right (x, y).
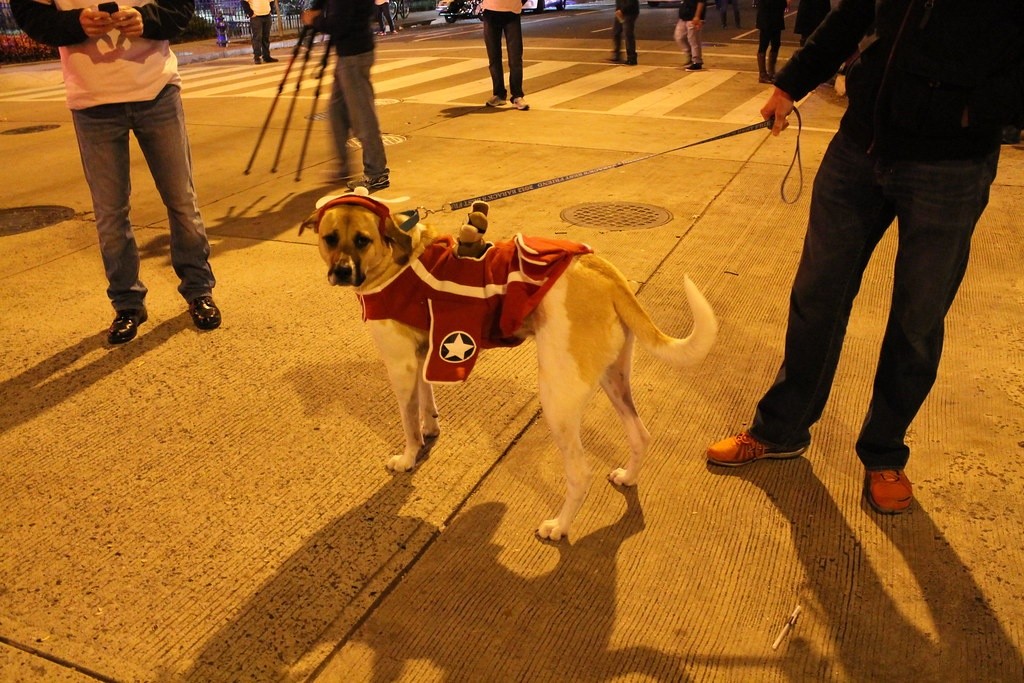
top-left (216, 14), bottom-right (231, 47)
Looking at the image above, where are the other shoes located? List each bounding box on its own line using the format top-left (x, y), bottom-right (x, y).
top-left (623, 60), bottom-right (637, 66)
top-left (378, 31), bottom-right (386, 35)
top-left (263, 57), bottom-right (278, 62)
top-left (254, 58), bottom-right (261, 64)
top-left (391, 31), bottom-right (398, 35)
top-left (682, 61), bottom-right (693, 66)
top-left (609, 57), bottom-right (620, 62)
top-left (686, 63), bottom-right (702, 72)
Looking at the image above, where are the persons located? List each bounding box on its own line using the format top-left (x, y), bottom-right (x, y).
top-left (376, 0), bottom-right (398, 36)
top-left (715, 0), bottom-right (742, 29)
top-left (753, 0), bottom-right (786, 83)
top-left (482, 0), bottom-right (529, 110)
top-left (9, 0), bottom-right (222, 343)
top-left (216, 13), bottom-right (228, 46)
top-left (674, 0), bottom-right (706, 71)
top-left (706, 0), bottom-right (1024, 512)
top-left (242, 0), bottom-right (278, 64)
top-left (607, 0), bottom-right (639, 65)
top-left (302, 0), bottom-right (390, 190)
top-left (793, 0), bottom-right (831, 47)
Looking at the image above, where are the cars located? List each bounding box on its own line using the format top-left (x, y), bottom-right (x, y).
top-left (436, 0), bottom-right (567, 23)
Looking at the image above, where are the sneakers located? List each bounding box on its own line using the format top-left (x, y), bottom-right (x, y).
top-left (706, 430), bottom-right (808, 466)
top-left (512, 97), bottom-right (529, 109)
top-left (329, 168), bottom-right (350, 180)
top-left (346, 169), bottom-right (390, 190)
top-left (868, 468), bottom-right (913, 513)
top-left (486, 95), bottom-right (506, 107)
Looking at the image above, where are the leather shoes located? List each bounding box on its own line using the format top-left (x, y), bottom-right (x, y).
top-left (107, 308), bottom-right (148, 344)
top-left (189, 296), bottom-right (221, 330)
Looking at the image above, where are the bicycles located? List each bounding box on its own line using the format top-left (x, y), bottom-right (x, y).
top-left (388, 0), bottom-right (409, 20)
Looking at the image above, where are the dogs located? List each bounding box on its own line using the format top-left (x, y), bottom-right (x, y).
top-left (298, 186), bottom-right (717, 542)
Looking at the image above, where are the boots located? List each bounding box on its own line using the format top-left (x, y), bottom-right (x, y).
top-left (768, 51), bottom-right (777, 78)
top-left (756, 52), bottom-right (774, 83)
